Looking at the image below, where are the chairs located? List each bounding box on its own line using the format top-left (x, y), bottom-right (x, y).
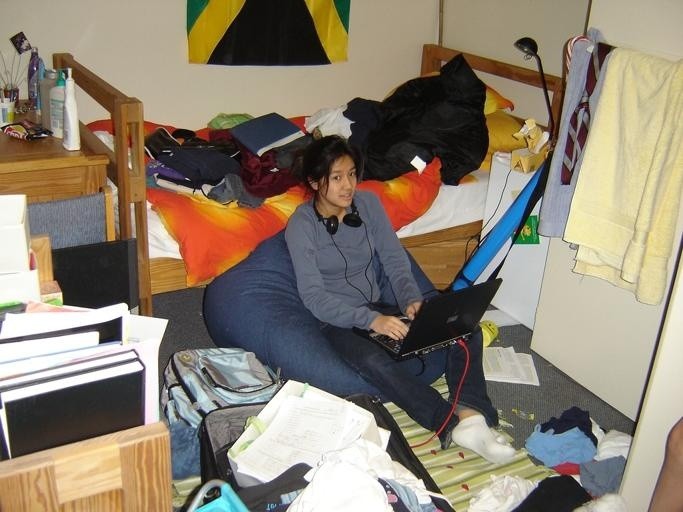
top-left (27, 185), bottom-right (116, 265)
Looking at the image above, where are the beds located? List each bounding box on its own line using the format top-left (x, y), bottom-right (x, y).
top-left (52, 44), bottom-right (566, 315)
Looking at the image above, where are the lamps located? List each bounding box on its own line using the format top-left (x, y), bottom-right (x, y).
top-left (514, 36), bottom-right (556, 140)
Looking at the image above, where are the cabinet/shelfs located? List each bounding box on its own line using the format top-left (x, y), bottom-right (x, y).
top-left (0, 234), bottom-right (173, 509)
top-left (1, 116), bottom-right (110, 201)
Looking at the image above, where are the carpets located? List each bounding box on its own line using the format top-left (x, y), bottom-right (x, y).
top-left (174, 368), bottom-right (558, 512)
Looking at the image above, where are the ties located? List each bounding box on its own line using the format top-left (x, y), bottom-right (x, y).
top-left (559, 41), bottom-right (615, 187)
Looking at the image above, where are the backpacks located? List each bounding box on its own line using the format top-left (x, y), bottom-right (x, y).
top-left (159, 346), bottom-right (283, 431)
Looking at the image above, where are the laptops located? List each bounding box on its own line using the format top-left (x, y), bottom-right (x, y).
top-left (353, 277), bottom-right (503, 362)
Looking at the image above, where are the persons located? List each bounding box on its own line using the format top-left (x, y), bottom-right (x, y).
top-left (15, 37), bottom-right (30, 54)
top-left (284, 133), bottom-right (515, 465)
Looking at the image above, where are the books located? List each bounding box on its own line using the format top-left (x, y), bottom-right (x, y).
top-left (0, 296), bottom-right (144, 459)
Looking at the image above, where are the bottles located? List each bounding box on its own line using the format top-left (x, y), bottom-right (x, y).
top-left (27, 47), bottom-right (65, 134)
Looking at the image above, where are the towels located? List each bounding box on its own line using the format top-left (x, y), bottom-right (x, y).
top-left (562, 49), bottom-right (683, 306)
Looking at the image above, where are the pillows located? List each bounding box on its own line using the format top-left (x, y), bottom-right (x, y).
top-left (473, 110), bottom-right (528, 170)
top-left (383, 70), bottom-right (513, 112)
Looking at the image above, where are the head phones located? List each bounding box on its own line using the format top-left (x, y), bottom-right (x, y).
top-left (313, 196), bottom-right (363, 235)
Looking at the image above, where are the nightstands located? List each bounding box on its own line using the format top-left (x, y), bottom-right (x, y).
top-left (479, 151), bottom-right (549, 330)
